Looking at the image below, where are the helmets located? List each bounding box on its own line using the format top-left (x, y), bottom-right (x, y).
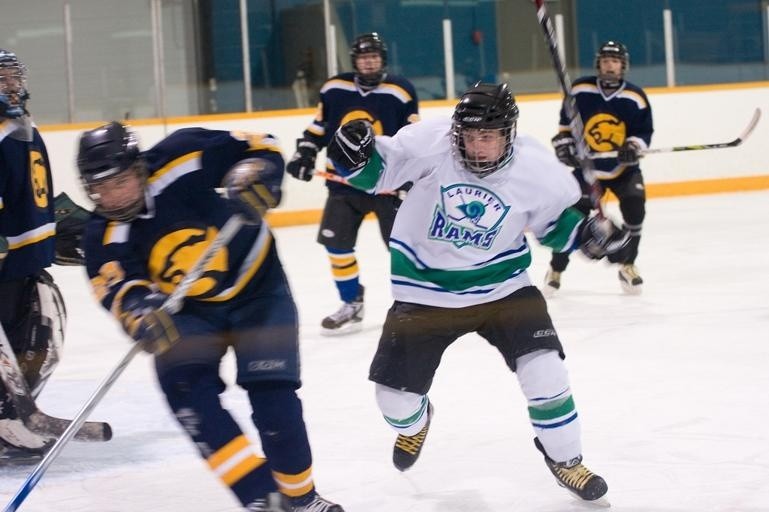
top-left (595, 42), bottom-right (629, 85)
top-left (449, 81), bottom-right (519, 177)
top-left (77, 123), bottom-right (148, 222)
top-left (1, 48), bottom-right (33, 142)
top-left (349, 32), bottom-right (390, 90)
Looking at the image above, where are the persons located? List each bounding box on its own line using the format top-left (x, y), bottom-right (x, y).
top-left (335, 81), bottom-right (633, 503)
top-left (283, 32), bottom-right (420, 332)
top-left (0, 47), bottom-right (60, 456)
top-left (540, 38), bottom-right (656, 292)
top-left (76, 116), bottom-right (344, 512)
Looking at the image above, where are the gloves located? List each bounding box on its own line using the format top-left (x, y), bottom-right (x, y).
top-left (219, 157), bottom-right (282, 225)
top-left (551, 131), bottom-right (579, 166)
top-left (285, 138), bottom-right (320, 182)
top-left (618, 141), bottom-right (641, 163)
top-left (110, 280), bottom-right (182, 354)
top-left (326, 119), bottom-right (375, 171)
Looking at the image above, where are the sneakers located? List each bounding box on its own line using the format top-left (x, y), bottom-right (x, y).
top-left (248, 491), bottom-right (282, 512)
top-left (281, 487), bottom-right (343, 512)
top-left (543, 270), bottom-right (561, 296)
top-left (320, 284), bottom-right (364, 335)
top-left (392, 398), bottom-right (434, 471)
top-left (534, 436), bottom-right (610, 509)
top-left (619, 267), bottom-right (643, 294)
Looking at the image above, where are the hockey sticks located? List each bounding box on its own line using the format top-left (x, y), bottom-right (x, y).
top-left (588, 108), bottom-right (760, 160)
top-left (1, 325), bottom-right (111, 442)
